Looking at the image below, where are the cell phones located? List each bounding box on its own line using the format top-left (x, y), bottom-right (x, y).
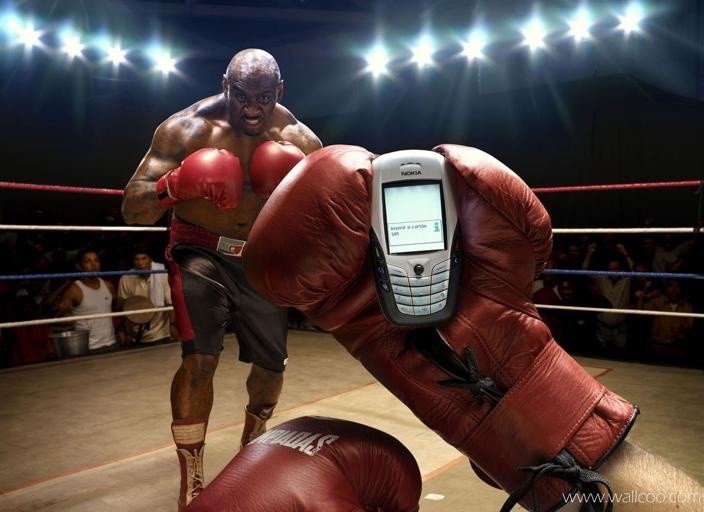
top-left (367, 148), bottom-right (464, 329)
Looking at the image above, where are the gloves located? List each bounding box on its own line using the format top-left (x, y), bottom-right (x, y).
top-left (248, 139), bottom-right (306, 199)
top-left (154, 146), bottom-right (245, 213)
top-left (170, 414), bottom-right (423, 512)
top-left (238, 143), bottom-right (642, 512)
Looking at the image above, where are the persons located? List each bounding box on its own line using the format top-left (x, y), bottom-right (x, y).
top-left (180, 140), bottom-right (704, 512)
top-left (119, 47), bottom-right (325, 512)
top-left (529, 215), bottom-right (704, 370)
top-left (1, 210), bottom-right (178, 367)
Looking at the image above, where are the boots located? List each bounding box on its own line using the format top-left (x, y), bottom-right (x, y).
top-left (240, 400), bottom-right (276, 452)
top-left (176, 439), bottom-right (209, 511)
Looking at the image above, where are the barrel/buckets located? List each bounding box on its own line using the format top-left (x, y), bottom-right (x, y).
top-left (49, 309), bottom-right (92, 358)
